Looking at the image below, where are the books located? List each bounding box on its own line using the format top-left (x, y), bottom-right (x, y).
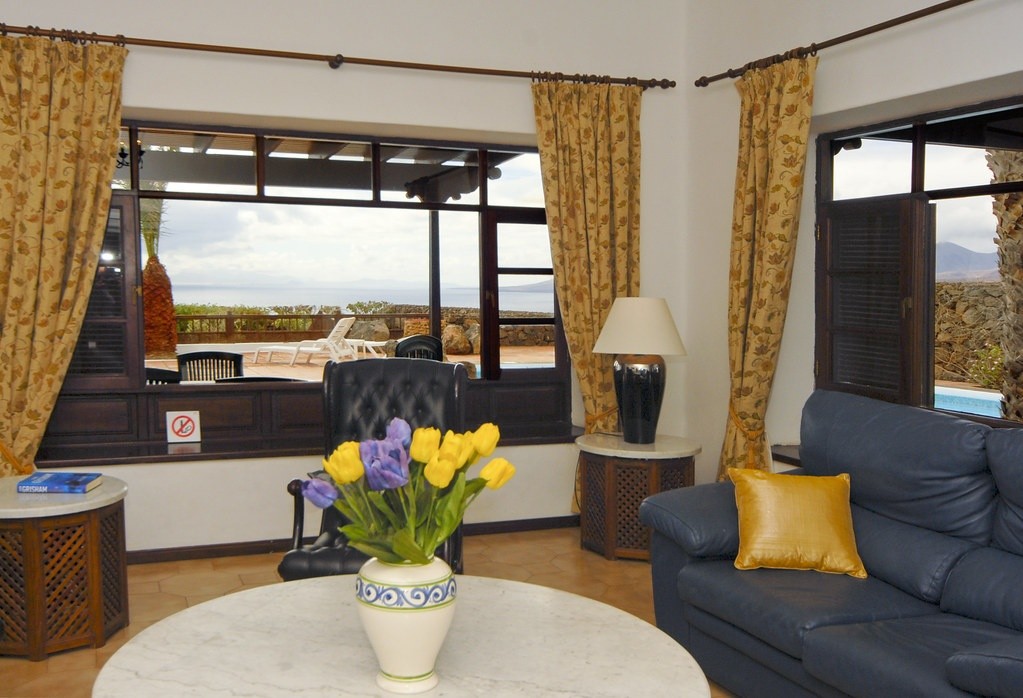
top-left (16, 470), bottom-right (101, 494)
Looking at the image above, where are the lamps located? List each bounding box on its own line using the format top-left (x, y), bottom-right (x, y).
top-left (589, 295), bottom-right (688, 444)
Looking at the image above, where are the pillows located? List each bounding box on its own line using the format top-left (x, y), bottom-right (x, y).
top-left (943, 639), bottom-right (1021, 698)
top-left (727, 468), bottom-right (867, 578)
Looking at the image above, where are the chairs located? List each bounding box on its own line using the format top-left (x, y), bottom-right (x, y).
top-left (214, 377), bottom-right (306, 382)
top-left (277, 356), bottom-right (469, 583)
top-left (146, 367), bottom-right (181, 385)
top-left (393, 335), bottom-right (444, 362)
top-left (176, 351), bottom-right (244, 381)
top-left (267, 316), bottom-right (359, 363)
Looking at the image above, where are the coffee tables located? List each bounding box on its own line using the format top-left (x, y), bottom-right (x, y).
top-left (90, 575), bottom-right (711, 698)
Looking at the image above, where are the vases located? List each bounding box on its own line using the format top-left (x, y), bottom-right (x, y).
top-left (354, 554), bottom-right (459, 696)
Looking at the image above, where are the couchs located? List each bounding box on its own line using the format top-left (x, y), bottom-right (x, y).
top-left (637, 388), bottom-right (1023, 698)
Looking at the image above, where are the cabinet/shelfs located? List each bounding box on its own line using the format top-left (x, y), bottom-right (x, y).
top-left (0, 470), bottom-right (132, 662)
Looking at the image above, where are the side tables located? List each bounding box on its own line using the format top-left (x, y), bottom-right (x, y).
top-left (576, 431), bottom-right (703, 563)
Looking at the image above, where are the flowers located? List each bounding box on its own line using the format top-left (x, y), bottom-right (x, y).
top-left (299, 416), bottom-right (517, 567)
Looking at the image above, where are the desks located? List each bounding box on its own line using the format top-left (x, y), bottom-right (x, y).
top-left (178, 377), bottom-right (323, 384)
